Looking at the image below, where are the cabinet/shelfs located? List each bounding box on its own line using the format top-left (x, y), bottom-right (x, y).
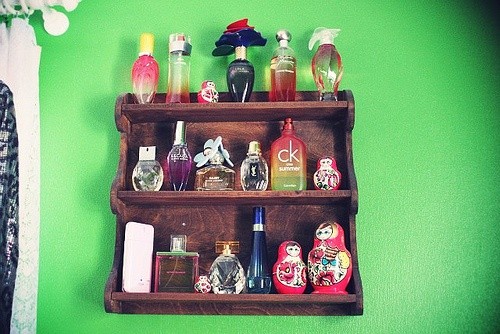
top-left (104, 89), bottom-right (364, 316)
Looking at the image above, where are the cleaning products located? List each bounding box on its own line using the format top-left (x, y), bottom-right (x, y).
top-left (270, 117), bottom-right (308, 191)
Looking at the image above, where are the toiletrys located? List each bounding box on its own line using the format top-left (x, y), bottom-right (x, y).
top-left (165, 32), bottom-right (193, 104)
top-left (130, 33), bottom-right (160, 104)
top-left (269, 29), bottom-right (298, 102)
top-left (308, 25), bottom-right (345, 102)
